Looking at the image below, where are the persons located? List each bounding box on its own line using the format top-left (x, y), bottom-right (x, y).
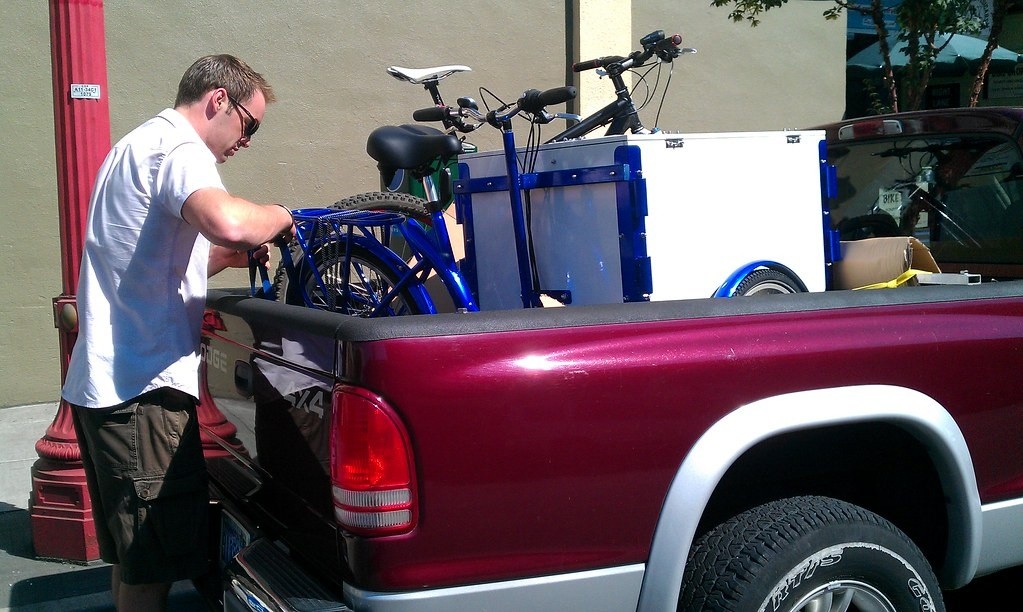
top-left (60, 55), bottom-right (296, 612)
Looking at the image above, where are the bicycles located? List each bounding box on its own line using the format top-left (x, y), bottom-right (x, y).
top-left (270, 29), bottom-right (843, 318)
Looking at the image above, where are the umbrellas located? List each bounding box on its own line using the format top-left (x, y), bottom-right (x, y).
top-left (846, 29), bottom-right (1023, 74)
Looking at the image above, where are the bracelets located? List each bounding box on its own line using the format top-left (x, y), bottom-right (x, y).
top-left (274, 203), bottom-right (294, 235)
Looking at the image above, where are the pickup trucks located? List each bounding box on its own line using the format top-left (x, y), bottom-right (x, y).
top-left (196, 107), bottom-right (1023, 611)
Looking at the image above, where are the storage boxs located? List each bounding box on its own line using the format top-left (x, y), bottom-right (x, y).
top-left (453, 129), bottom-right (830, 311)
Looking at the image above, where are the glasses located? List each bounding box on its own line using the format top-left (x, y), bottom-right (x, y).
top-left (227, 94), bottom-right (260, 136)
